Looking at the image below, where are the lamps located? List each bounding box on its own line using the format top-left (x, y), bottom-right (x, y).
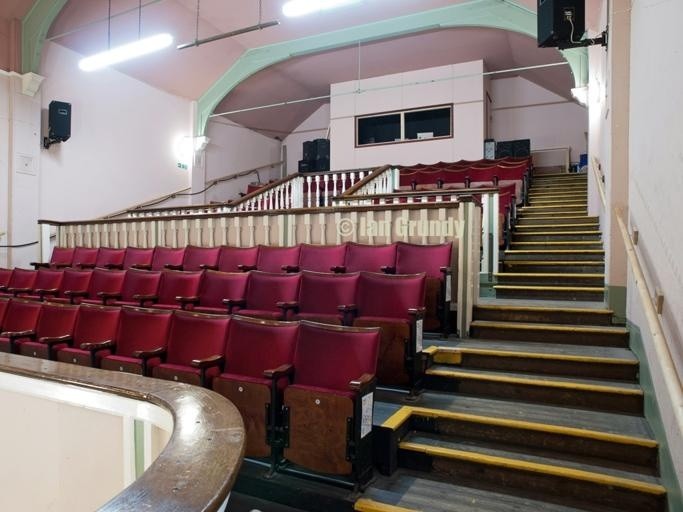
top-left (78, 0), bottom-right (175, 75)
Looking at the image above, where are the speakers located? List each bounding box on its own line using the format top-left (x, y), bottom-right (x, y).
top-left (536, 1), bottom-right (585, 48)
top-left (48, 100), bottom-right (72, 138)
top-left (497, 140), bottom-right (513, 157)
top-left (512, 139), bottom-right (530, 158)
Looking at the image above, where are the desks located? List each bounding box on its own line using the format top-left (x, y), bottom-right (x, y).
top-left (529, 146), bottom-right (570, 174)
